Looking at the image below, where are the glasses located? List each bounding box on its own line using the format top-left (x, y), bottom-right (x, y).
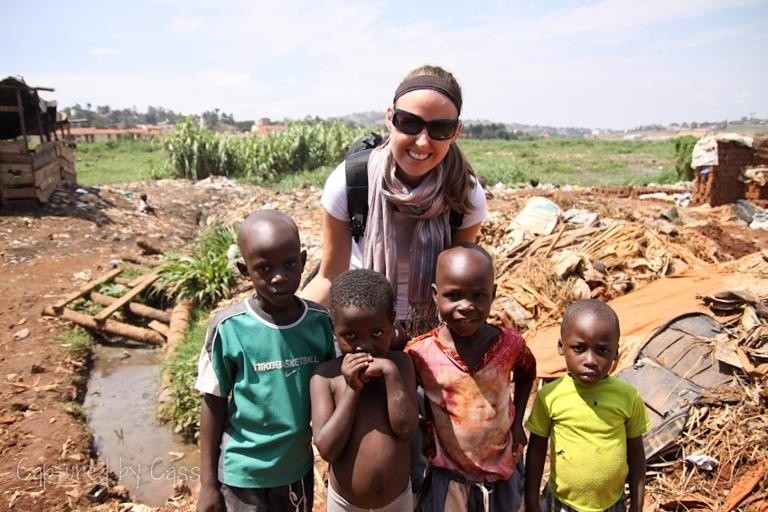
top-left (391, 105), bottom-right (460, 141)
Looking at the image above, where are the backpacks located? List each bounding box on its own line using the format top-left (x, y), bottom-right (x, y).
top-left (346, 133), bottom-right (467, 243)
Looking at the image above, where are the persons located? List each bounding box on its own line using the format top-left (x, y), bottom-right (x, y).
top-left (522, 298), bottom-right (651, 512)
top-left (404, 243), bottom-right (536, 512)
top-left (307, 268), bottom-right (421, 512)
top-left (297, 63), bottom-right (487, 336)
top-left (194, 210), bottom-right (344, 512)
